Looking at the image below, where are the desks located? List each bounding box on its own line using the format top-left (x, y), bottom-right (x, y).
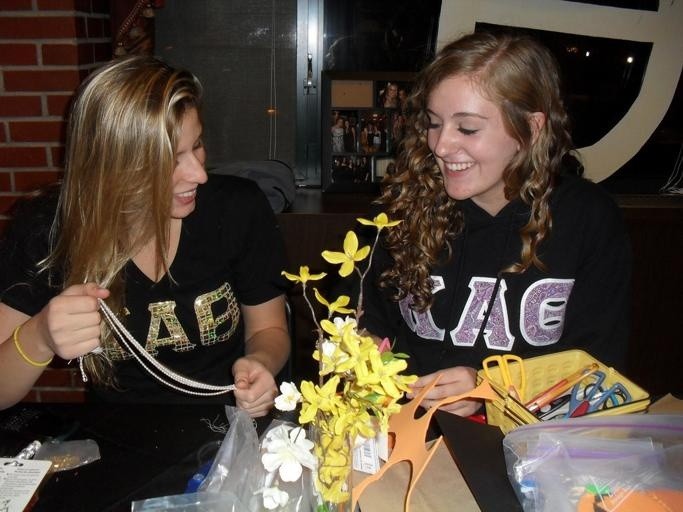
top-left (0, 400), bottom-right (524, 512)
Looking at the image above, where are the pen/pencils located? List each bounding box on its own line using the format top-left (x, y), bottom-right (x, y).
top-left (525, 363), bottom-right (598, 414)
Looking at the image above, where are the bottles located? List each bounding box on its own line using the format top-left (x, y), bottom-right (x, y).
top-left (330, 110), bottom-right (404, 152)
top-left (331, 155), bottom-right (370, 183)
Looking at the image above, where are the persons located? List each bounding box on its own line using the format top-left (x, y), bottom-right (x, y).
top-left (330, 80), bottom-right (409, 186)
top-left (1, 58), bottom-right (291, 420)
top-left (338, 33), bottom-right (636, 417)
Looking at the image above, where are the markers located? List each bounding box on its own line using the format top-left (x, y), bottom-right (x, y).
top-left (15, 436), bottom-right (45, 458)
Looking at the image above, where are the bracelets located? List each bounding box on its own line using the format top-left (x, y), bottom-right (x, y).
top-left (12, 326), bottom-right (53, 369)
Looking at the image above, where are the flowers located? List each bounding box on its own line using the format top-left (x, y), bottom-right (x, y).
top-left (253, 214), bottom-right (417, 512)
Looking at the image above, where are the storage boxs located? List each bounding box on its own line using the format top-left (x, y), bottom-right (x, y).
top-left (476, 349), bottom-right (650, 436)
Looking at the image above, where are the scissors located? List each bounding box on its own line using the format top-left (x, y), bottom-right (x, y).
top-left (563, 370), bottom-right (632, 419)
top-left (481, 355), bottom-right (526, 403)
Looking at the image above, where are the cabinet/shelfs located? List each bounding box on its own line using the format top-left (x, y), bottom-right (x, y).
top-left (321, 70), bottom-right (418, 193)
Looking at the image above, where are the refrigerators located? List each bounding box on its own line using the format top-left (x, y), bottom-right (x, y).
top-left (149, 0), bottom-right (322, 215)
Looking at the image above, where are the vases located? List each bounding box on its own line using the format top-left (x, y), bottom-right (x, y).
top-left (309, 422), bottom-right (353, 512)
